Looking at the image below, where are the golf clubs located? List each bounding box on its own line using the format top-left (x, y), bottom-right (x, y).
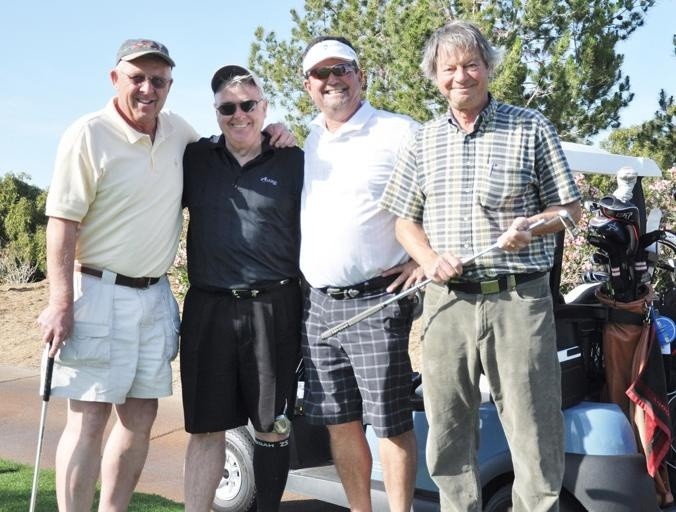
top-left (582, 252), bottom-right (613, 301)
top-left (322, 218), bottom-right (573, 354)
top-left (321, 211), bottom-right (575, 341)
top-left (29, 342), bottom-right (53, 511)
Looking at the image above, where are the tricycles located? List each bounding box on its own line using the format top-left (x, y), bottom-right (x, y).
top-left (186, 167), bottom-right (675, 510)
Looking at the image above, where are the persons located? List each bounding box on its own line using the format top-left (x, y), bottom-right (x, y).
top-left (377, 22), bottom-right (581, 512)
top-left (39, 38), bottom-right (294, 511)
top-left (182, 63), bottom-right (304, 512)
top-left (298, 37), bottom-right (426, 512)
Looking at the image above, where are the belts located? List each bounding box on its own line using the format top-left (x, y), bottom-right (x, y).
top-left (447, 271), bottom-right (548, 294)
top-left (319, 275), bottom-right (398, 300)
top-left (72, 265), bottom-right (159, 290)
top-left (193, 278), bottom-right (290, 301)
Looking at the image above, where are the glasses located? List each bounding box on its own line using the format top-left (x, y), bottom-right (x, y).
top-left (305, 64), bottom-right (357, 79)
top-left (120, 71), bottom-right (171, 88)
top-left (214, 98), bottom-right (264, 115)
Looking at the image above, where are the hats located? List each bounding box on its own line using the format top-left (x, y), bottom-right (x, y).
top-left (211, 65), bottom-right (259, 91)
top-left (116, 39), bottom-right (175, 68)
top-left (303, 40), bottom-right (360, 76)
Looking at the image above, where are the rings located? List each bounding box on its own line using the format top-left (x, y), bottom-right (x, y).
top-left (287, 129), bottom-right (293, 135)
